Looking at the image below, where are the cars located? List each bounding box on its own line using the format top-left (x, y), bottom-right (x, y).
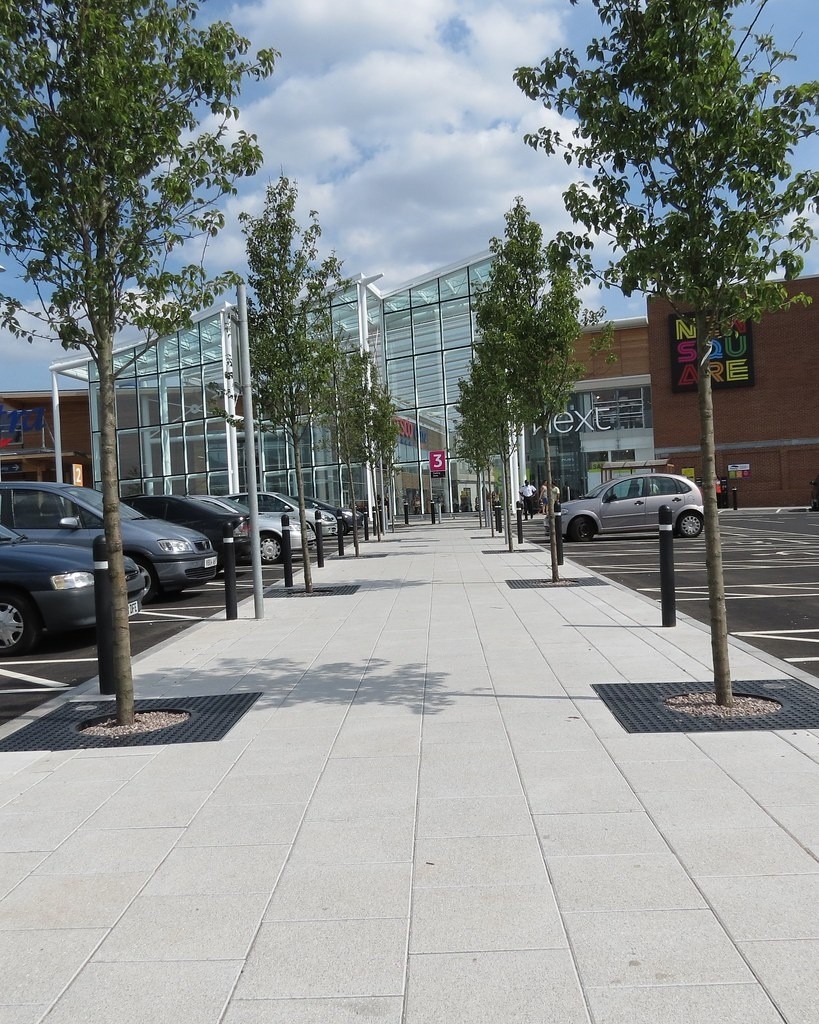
top-left (0, 525), bottom-right (146, 657)
top-left (186, 496), bottom-right (316, 565)
top-left (223, 492), bottom-right (338, 537)
top-left (288, 496), bottom-right (364, 536)
top-left (809, 475), bottom-right (819, 511)
top-left (120, 494), bottom-right (250, 572)
top-left (0, 481), bottom-right (218, 605)
top-left (542, 472), bottom-right (705, 543)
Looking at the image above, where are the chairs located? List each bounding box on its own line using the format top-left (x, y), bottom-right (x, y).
top-left (628, 483), bottom-right (639, 498)
top-left (41, 499), bottom-right (60, 529)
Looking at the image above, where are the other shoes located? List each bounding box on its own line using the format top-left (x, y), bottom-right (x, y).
top-left (531, 514), bottom-right (533, 519)
top-left (542, 513), bottom-right (547, 515)
top-left (523, 518), bottom-right (528, 520)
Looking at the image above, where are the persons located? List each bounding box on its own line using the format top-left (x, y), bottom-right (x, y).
top-left (716, 481), bottom-right (721, 509)
top-left (519, 479), bottom-right (537, 521)
top-left (650, 480), bottom-right (658, 493)
top-left (538, 480), bottom-right (548, 515)
top-left (414, 496), bottom-right (420, 515)
top-left (552, 482), bottom-right (560, 504)
